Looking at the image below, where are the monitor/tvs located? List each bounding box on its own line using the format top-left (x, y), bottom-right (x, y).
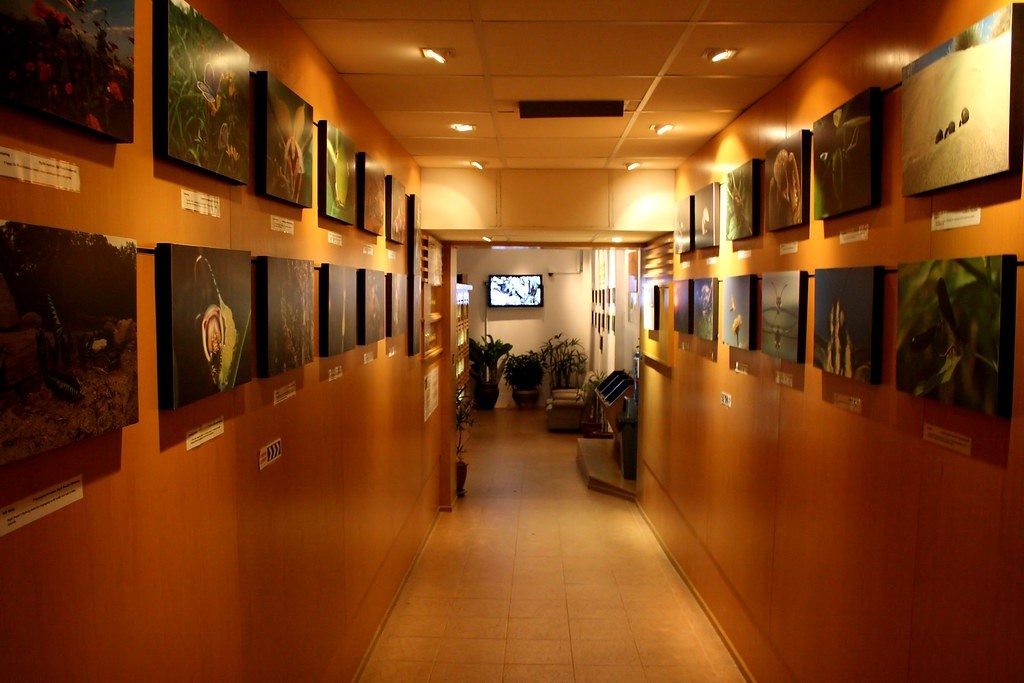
top-left (488, 274), bottom-right (544, 307)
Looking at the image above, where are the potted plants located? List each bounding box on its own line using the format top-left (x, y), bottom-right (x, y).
top-left (469, 335), bottom-right (513, 410)
top-left (539, 332), bottom-right (588, 398)
top-left (503, 350), bottom-right (544, 410)
top-left (456, 388), bottom-right (481, 498)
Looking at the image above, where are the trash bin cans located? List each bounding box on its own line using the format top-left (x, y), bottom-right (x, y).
top-left (616, 395), bottom-right (637, 481)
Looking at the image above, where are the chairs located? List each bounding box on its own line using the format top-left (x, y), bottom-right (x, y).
top-left (545, 376), bottom-right (600, 432)
top-left (546, 371), bottom-right (595, 407)
